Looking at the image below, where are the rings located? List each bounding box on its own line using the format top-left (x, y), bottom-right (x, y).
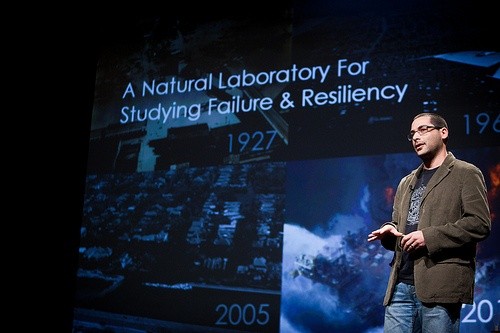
top-left (411, 244), bottom-right (414, 248)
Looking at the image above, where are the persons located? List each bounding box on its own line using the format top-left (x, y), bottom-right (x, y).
top-left (367, 113), bottom-right (493, 333)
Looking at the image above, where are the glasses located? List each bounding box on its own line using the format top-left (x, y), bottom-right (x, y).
top-left (408, 125), bottom-right (439, 141)
